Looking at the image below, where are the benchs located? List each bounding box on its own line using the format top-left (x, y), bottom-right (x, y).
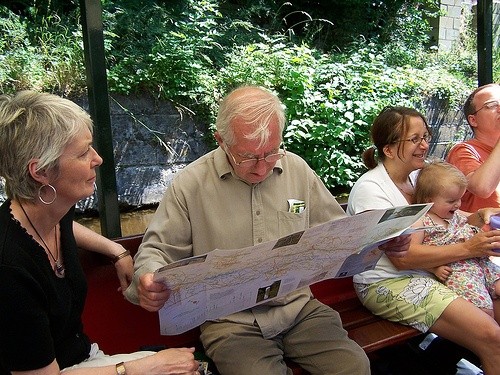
top-left (81, 233), bottom-right (426, 375)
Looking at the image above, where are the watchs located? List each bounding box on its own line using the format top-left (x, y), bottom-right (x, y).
top-left (115, 361), bottom-right (127, 375)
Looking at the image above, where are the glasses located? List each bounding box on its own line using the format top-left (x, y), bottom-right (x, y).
top-left (392, 135), bottom-right (432, 145)
top-left (224, 139), bottom-right (286, 168)
top-left (472, 100), bottom-right (500, 115)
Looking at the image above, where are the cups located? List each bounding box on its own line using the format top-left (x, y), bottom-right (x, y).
top-left (489, 216), bottom-right (500, 253)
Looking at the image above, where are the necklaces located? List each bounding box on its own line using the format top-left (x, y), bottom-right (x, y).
top-left (18, 200), bottom-right (65, 276)
top-left (382, 162), bottom-right (416, 196)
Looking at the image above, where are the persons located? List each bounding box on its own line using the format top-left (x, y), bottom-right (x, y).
top-left (346, 106), bottom-right (500, 375)
top-left (0, 90), bottom-right (201, 375)
top-left (446, 83), bottom-right (500, 232)
top-left (124, 86), bottom-right (412, 375)
top-left (408, 160), bottom-right (500, 319)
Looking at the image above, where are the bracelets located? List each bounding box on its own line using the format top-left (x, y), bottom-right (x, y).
top-left (477, 209), bottom-right (485, 222)
top-left (111, 249), bottom-right (131, 265)
